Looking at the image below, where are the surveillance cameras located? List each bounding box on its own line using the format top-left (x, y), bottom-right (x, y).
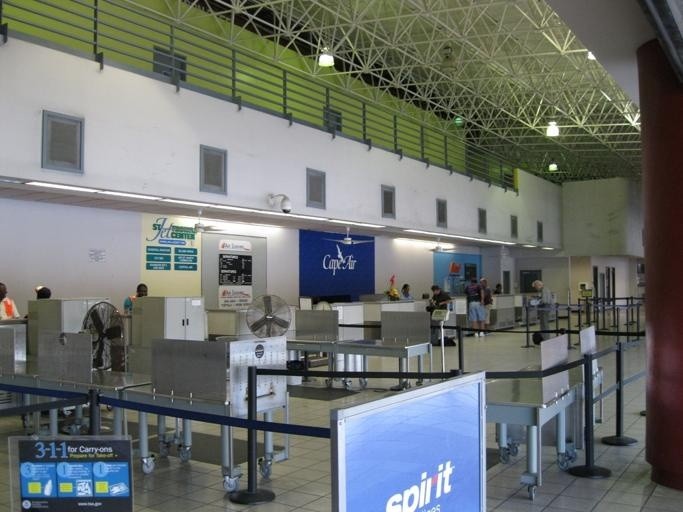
top-left (281, 197), bottom-right (291, 213)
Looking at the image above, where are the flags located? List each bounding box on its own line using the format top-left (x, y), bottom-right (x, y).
top-left (448, 261), bottom-right (461, 273)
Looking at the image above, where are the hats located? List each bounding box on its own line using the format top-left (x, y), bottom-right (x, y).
top-left (35, 287), bottom-right (51, 298)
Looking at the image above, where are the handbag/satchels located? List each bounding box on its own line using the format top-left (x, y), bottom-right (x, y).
top-left (532, 333), bottom-right (543, 344)
top-left (485, 294), bottom-right (491, 305)
top-left (439, 337), bottom-right (456, 346)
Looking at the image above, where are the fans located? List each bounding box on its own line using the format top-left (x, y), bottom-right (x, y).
top-left (246, 294), bottom-right (293, 338)
top-left (321, 227), bottom-right (377, 246)
top-left (80, 301), bottom-right (127, 370)
top-left (174, 211), bottom-right (225, 234)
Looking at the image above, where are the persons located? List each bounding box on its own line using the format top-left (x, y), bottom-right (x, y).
top-left (0, 281), bottom-right (20, 319)
top-left (464, 278), bottom-right (486, 336)
top-left (492, 283), bottom-right (502, 294)
top-left (429, 284), bottom-right (452, 309)
top-left (479, 278), bottom-right (495, 335)
top-left (400, 284), bottom-right (413, 299)
top-left (122, 284), bottom-right (148, 312)
top-left (531, 280), bottom-right (552, 339)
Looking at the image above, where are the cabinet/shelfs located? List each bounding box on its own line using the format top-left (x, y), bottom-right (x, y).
top-left (131, 296), bottom-right (206, 341)
top-left (27, 298), bottom-right (97, 358)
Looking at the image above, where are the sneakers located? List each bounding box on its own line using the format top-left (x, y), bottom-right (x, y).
top-left (475, 332), bottom-right (488, 337)
